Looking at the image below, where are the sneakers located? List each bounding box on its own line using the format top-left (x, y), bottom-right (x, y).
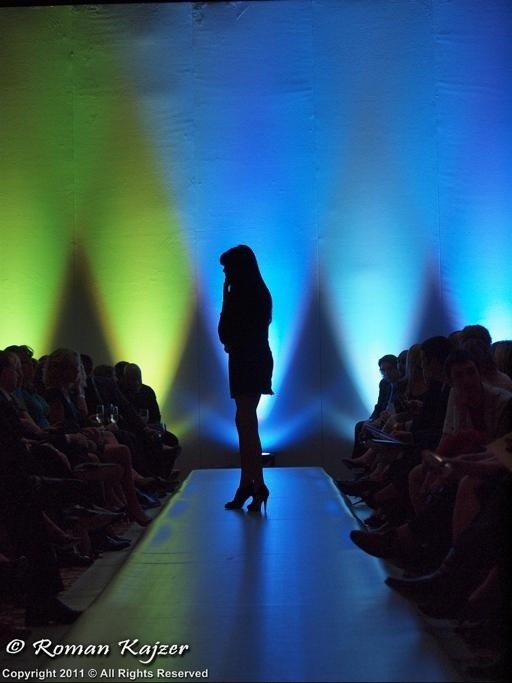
top-left (338, 457), bottom-right (400, 529)
top-left (90, 512), bottom-right (124, 529)
top-left (129, 509), bottom-right (152, 527)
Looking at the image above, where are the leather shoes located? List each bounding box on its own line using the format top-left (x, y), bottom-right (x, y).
top-left (350, 529), bottom-right (425, 573)
top-left (94, 536), bottom-right (132, 551)
top-left (24, 599), bottom-right (83, 625)
top-left (138, 494), bottom-right (163, 508)
top-left (385, 568), bottom-right (466, 602)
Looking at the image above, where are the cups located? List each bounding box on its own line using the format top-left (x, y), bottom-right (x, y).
top-left (110, 406), bottom-right (119, 423)
top-left (139, 409), bottom-right (148, 423)
top-left (96, 405), bottom-right (105, 423)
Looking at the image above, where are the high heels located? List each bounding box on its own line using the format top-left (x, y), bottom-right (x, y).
top-left (225, 484), bottom-right (268, 513)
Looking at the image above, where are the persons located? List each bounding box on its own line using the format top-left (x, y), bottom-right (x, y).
top-left (217, 245), bottom-right (275, 510)
top-left (2, 343), bottom-right (183, 649)
top-left (337, 324), bottom-right (512, 683)
